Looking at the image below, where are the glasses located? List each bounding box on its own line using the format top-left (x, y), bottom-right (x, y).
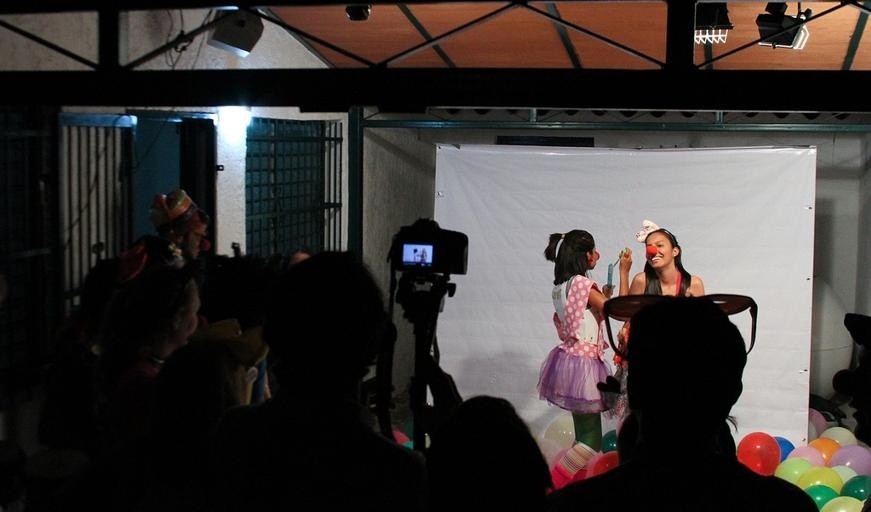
top-left (753, 0), bottom-right (813, 50)
top-left (206, 6), bottom-right (270, 58)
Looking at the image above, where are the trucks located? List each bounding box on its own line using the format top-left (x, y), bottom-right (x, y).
top-left (736, 426), bottom-right (871, 512)
top-left (598, 430), bottom-right (618, 452)
top-left (593, 451), bottom-right (618, 477)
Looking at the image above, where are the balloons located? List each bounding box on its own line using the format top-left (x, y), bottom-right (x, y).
top-left (598, 430), bottom-right (618, 452)
top-left (736, 426), bottom-right (871, 512)
top-left (593, 451), bottom-right (618, 477)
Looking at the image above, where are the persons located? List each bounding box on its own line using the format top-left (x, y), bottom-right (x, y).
top-left (1, 190), bottom-right (267, 509)
top-left (209, 250), bottom-right (558, 511)
top-left (534, 293), bottom-right (819, 509)
top-left (614, 220), bottom-right (707, 367)
top-left (535, 229), bottom-right (634, 492)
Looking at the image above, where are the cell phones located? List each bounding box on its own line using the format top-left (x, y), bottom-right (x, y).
top-left (389, 217), bottom-right (468, 274)
